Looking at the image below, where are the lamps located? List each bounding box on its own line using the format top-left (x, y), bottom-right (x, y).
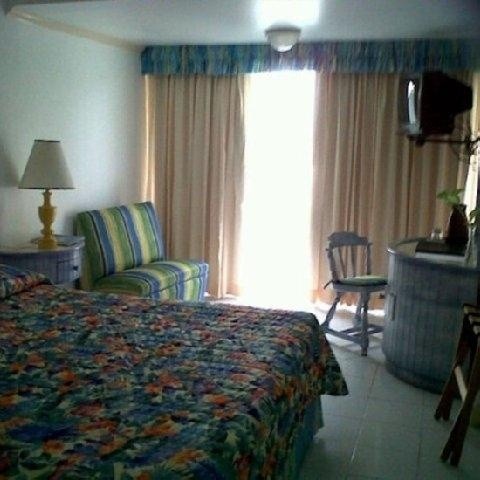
top-left (18, 139), bottom-right (77, 245)
top-left (264, 26), bottom-right (301, 52)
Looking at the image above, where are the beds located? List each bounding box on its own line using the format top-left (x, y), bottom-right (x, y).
top-left (0, 263), bottom-right (322, 480)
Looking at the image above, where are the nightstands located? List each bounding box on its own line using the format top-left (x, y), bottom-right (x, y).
top-left (0, 240), bottom-right (85, 291)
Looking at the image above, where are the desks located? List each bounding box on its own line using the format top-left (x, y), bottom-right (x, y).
top-left (382, 237), bottom-right (478, 398)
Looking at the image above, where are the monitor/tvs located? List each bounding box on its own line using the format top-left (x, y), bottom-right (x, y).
top-left (395, 69), bottom-right (473, 136)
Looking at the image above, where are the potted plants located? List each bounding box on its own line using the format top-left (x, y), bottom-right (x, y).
top-left (433, 187), bottom-right (480, 269)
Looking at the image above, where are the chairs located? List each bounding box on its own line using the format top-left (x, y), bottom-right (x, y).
top-left (320, 231), bottom-right (388, 356)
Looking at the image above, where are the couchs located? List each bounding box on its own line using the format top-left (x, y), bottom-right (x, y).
top-left (73, 201), bottom-right (210, 300)
